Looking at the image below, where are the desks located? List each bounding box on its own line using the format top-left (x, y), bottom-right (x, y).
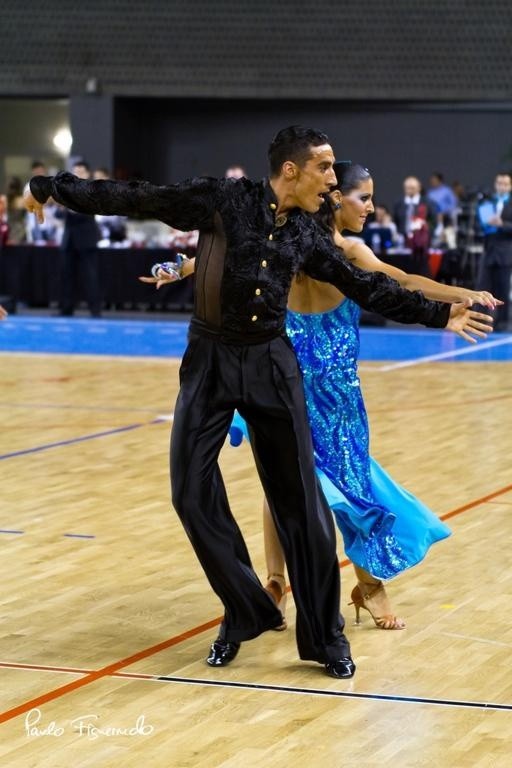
top-left (0, 247), bottom-right (512, 320)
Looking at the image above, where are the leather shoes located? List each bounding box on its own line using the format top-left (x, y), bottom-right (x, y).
top-left (325, 656), bottom-right (355, 679)
top-left (206, 637), bottom-right (240, 666)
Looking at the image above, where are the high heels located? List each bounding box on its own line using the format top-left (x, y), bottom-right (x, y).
top-left (267, 573), bottom-right (287, 612)
top-left (351, 581), bottom-right (406, 629)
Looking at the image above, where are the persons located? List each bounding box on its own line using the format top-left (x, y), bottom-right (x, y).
top-left (1, 147), bottom-right (511, 338)
top-left (139, 159), bottom-right (506, 631)
top-left (15, 120), bottom-right (498, 682)
